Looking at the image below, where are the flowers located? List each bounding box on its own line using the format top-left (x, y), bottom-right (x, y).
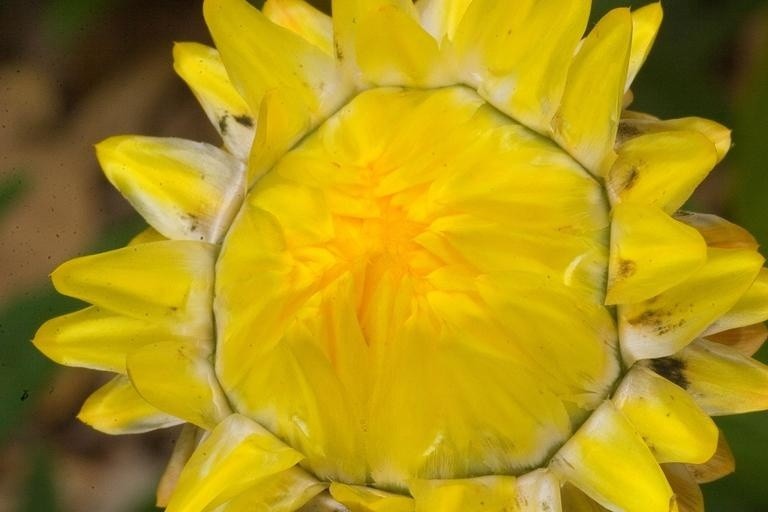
top-left (26, 2), bottom-right (768, 512)
